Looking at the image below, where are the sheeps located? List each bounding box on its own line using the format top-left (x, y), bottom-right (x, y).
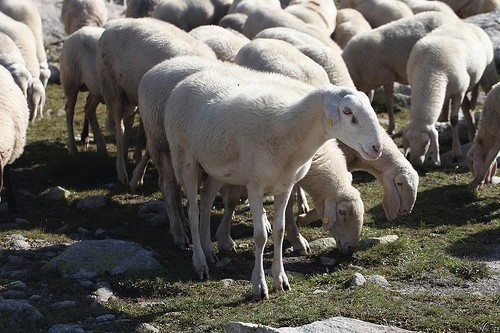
top-left (0, 0), bottom-right (500, 300)
